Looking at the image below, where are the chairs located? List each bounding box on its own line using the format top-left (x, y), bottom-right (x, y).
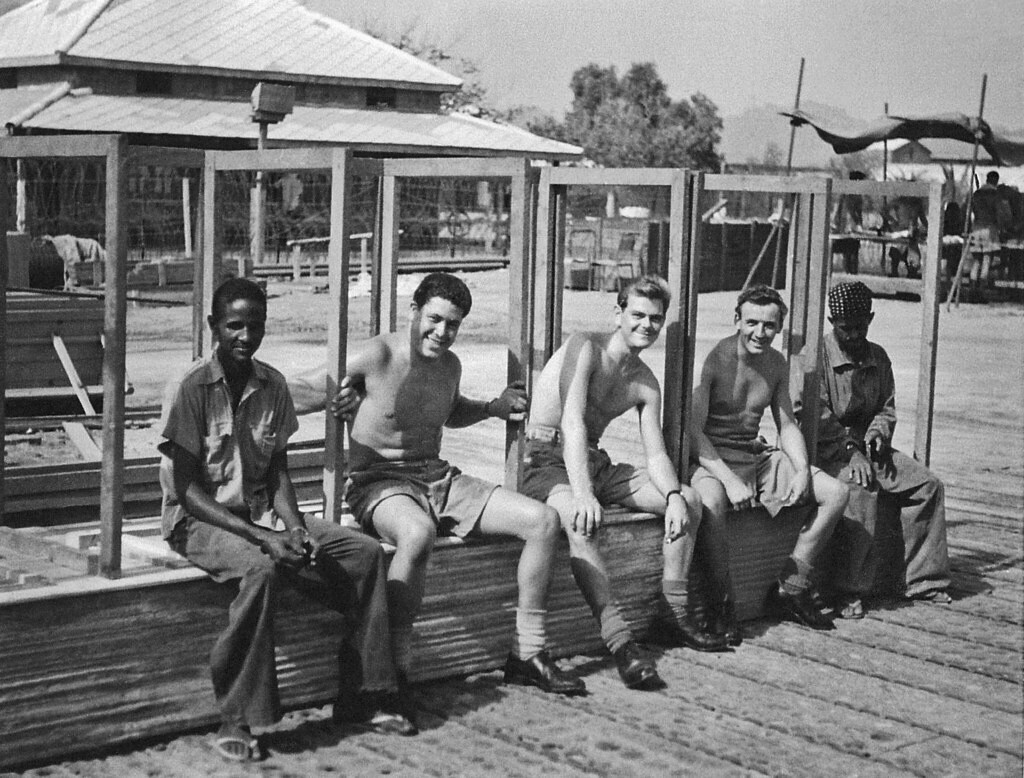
top-left (588, 232), bottom-right (637, 295)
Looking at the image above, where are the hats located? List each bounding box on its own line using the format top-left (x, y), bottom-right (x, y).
top-left (828, 281), bottom-right (873, 318)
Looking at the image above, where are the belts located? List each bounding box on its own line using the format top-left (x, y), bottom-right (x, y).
top-left (526, 424), bottom-right (599, 450)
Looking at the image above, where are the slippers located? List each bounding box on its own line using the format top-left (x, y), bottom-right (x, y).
top-left (834, 591), bottom-right (864, 619)
top-left (215, 736), bottom-right (260, 761)
top-left (332, 705), bottom-right (416, 737)
top-left (912, 587), bottom-right (953, 603)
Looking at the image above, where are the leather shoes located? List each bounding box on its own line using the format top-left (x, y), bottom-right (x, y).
top-left (614, 640), bottom-right (658, 688)
top-left (382, 686), bottom-right (444, 730)
top-left (764, 581), bottom-right (835, 630)
top-left (706, 599), bottom-right (741, 645)
top-left (653, 615), bottom-right (730, 651)
top-left (502, 649), bottom-right (586, 693)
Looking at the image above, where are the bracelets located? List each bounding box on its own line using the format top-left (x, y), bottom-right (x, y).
top-left (666, 490), bottom-right (685, 506)
top-left (847, 444), bottom-right (853, 450)
top-left (485, 402), bottom-right (489, 416)
top-left (291, 527), bottom-right (305, 534)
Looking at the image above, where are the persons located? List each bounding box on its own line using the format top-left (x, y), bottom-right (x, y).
top-left (942, 201), bottom-right (963, 281)
top-left (881, 196), bottom-right (928, 277)
top-left (836, 172), bottom-right (865, 274)
top-left (288, 273), bottom-right (587, 727)
top-left (517, 271), bottom-right (728, 686)
top-left (969, 171), bottom-right (1003, 303)
top-left (275, 172), bottom-right (303, 214)
top-left (156, 278), bottom-right (415, 762)
top-left (689, 286), bottom-right (849, 646)
top-left (791, 281), bottom-right (953, 619)
